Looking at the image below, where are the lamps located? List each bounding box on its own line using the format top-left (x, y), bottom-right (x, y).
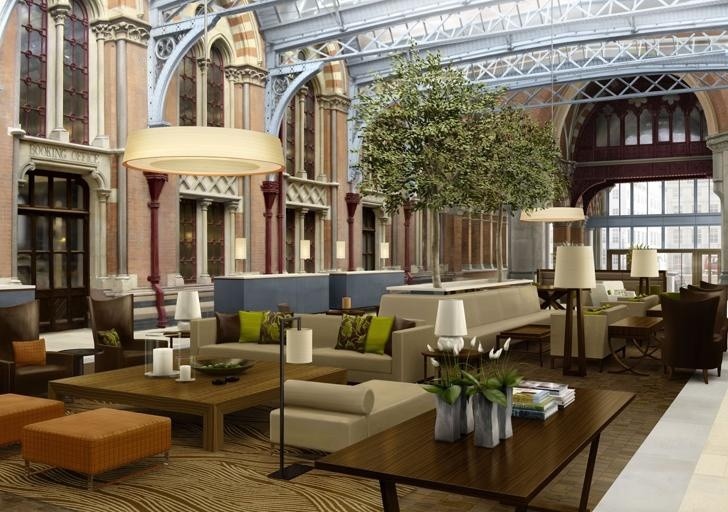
top-left (520, 2), bottom-right (586, 224)
top-left (173, 290), bottom-right (202, 321)
top-left (276, 316), bottom-right (313, 478)
top-left (630, 248), bottom-right (659, 295)
top-left (123, 0), bottom-right (287, 180)
top-left (553, 244), bottom-right (596, 376)
top-left (431, 298), bottom-right (468, 353)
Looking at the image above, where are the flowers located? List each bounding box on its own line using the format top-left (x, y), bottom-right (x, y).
top-left (421, 334), bottom-right (525, 404)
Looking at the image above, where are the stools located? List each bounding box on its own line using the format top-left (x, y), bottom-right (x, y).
top-left (0, 392), bottom-right (173, 495)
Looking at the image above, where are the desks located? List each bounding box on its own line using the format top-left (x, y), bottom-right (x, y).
top-left (538, 288), bottom-right (566, 309)
top-left (314, 376), bottom-right (634, 512)
top-left (496, 323), bottom-right (551, 366)
top-left (608, 302), bottom-right (664, 377)
top-left (48, 357), bottom-right (347, 454)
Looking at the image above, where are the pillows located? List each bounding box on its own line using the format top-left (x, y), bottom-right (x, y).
top-left (335, 314), bottom-right (372, 354)
top-left (11, 339), bottom-right (47, 367)
top-left (96, 327), bottom-right (121, 348)
top-left (365, 315), bottom-right (396, 355)
top-left (283, 381), bottom-right (374, 414)
top-left (213, 309), bottom-right (295, 344)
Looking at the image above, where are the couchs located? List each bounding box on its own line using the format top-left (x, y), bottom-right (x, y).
top-left (616, 292), bottom-right (660, 319)
top-left (550, 303), bottom-right (629, 371)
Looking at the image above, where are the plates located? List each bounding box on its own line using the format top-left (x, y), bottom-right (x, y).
top-left (180, 357), bottom-right (258, 376)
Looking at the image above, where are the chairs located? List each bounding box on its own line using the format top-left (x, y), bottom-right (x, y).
top-left (89, 293), bottom-right (168, 374)
top-left (660, 280), bottom-right (728, 385)
top-left (0, 299), bottom-right (84, 401)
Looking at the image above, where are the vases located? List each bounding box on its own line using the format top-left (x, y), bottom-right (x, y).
top-left (435, 385), bottom-right (514, 450)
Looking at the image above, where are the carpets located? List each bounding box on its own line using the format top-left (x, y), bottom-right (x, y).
top-left (1, 394), bottom-right (417, 512)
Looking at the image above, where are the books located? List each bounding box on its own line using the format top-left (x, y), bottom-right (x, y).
top-left (512, 380), bottom-right (576, 421)
